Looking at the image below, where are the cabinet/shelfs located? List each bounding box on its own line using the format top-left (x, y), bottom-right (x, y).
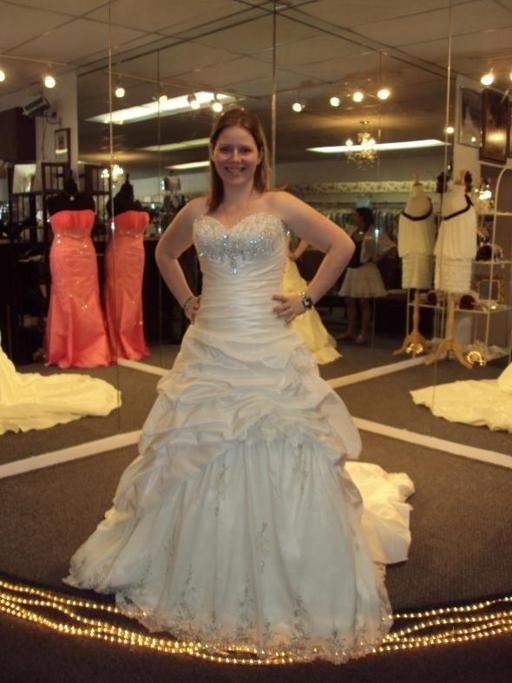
top-left (438, 166), bottom-right (512, 366)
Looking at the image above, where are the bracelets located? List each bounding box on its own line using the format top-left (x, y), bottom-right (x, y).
top-left (181, 296), bottom-right (197, 308)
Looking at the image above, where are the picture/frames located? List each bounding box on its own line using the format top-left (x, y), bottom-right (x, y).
top-left (40, 128), bottom-right (71, 194)
top-left (456, 86), bottom-right (510, 166)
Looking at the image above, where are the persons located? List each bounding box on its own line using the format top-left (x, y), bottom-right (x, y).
top-left (401, 171), bottom-right (480, 310)
top-left (337, 207), bottom-right (397, 344)
top-left (281, 186), bottom-right (343, 366)
top-left (62, 108), bottom-right (414, 666)
top-left (46, 180), bottom-right (112, 370)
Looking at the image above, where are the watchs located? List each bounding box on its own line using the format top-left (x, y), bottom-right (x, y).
top-left (300, 292), bottom-right (315, 310)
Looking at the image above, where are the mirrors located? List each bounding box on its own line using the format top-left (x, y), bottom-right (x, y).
top-left (2, 0), bottom-right (456, 468)
